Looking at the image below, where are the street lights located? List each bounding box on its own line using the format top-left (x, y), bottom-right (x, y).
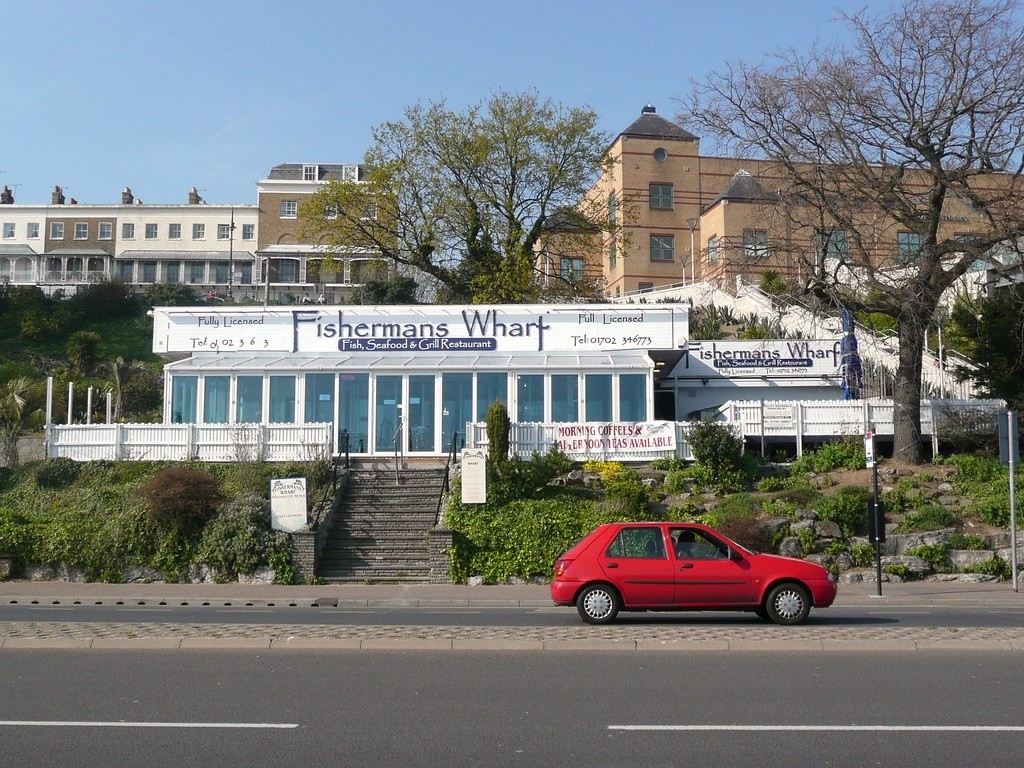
top-left (688, 215), bottom-right (700, 284)
top-left (681, 253), bottom-right (689, 286)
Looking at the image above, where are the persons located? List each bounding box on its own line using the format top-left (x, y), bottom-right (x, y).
top-left (935, 344), bottom-right (947, 371)
top-left (302, 293), bottom-right (312, 302)
top-left (676, 531), bottom-right (696, 559)
top-left (318, 293), bottom-right (326, 305)
top-left (206, 290), bottom-right (214, 301)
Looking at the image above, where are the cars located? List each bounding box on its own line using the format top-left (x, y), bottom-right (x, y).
top-left (550, 524), bottom-right (839, 627)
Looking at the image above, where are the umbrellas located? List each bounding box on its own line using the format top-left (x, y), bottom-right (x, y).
top-left (840, 307), bottom-right (863, 400)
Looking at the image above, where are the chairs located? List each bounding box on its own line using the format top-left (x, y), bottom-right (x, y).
top-left (645, 540), bottom-right (658, 557)
